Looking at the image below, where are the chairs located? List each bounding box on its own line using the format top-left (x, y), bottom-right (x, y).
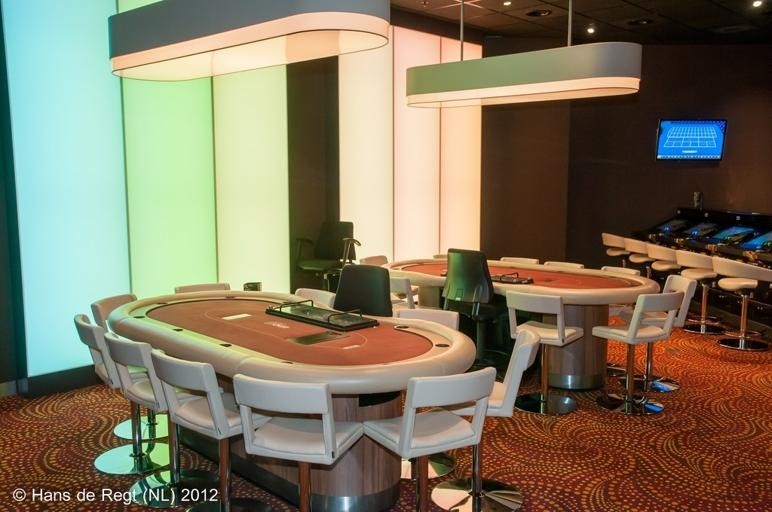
top-left (617, 273), bottom-right (697, 393)
top-left (102, 332), bottom-right (231, 507)
top-left (290, 219), bottom-right (362, 291)
top-left (297, 285), bottom-right (335, 311)
top-left (337, 265), bottom-right (391, 315)
top-left (151, 349), bottom-right (275, 512)
top-left (712, 255), bottom-right (772, 353)
top-left (391, 276), bottom-right (418, 309)
top-left (364, 366), bottom-right (498, 511)
top-left (231, 373), bottom-right (364, 512)
top-left (601, 232), bottom-right (628, 269)
top-left (429, 329), bottom-right (543, 512)
top-left (590, 290), bottom-right (686, 419)
top-left (362, 254), bottom-right (389, 267)
top-left (675, 248), bottom-right (725, 334)
top-left (74, 313), bottom-right (176, 474)
top-left (386, 310), bottom-right (461, 480)
top-left (600, 264), bottom-right (641, 377)
top-left (646, 242), bottom-right (682, 321)
top-left (624, 237), bottom-right (651, 278)
top-left (506, 290), bottom-right (587, 416)
top-left (90, 292), bottom-right (171, 440)
top-left (544, 259), bottom-right (584, 269)
top-left (174, 282), bottom-right (229, 291)
top-left (442, 248), bottom-right (507, 371)
top-left (501, 255), bottom-right (540, 265)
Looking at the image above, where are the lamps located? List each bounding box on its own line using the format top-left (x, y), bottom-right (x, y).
top-left (107, 2), bottom-right (392, 82)
top-left (406, 0), bottom-right (644, 107)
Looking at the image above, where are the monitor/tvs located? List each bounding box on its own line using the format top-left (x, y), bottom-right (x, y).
top-left (654, 115), bottom-right (728, 169)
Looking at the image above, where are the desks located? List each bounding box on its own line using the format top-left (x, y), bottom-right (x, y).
top-left (105, 288), bottom-right (477, 512)
top-left (385, 257), bottom-right (659, 393)
